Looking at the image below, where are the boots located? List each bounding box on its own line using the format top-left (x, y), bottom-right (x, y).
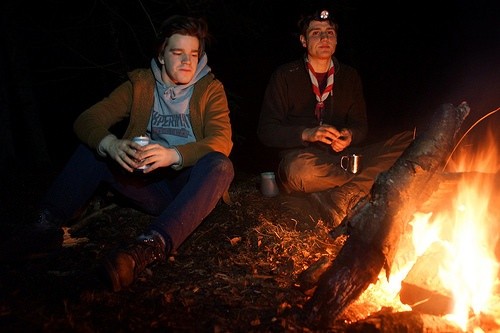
top-left (1, 209), bottom-right (71, 261)
top-left (106, 238), bottom-right (163, 291)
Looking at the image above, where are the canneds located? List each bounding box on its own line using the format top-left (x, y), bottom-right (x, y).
top-left (133, 136), bottom-right (151, 169)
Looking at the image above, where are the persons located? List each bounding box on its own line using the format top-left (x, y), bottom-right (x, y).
top-left (258, 9), bottom-right (418, 234)
top-left (37, 13), bottom-right (235, 293)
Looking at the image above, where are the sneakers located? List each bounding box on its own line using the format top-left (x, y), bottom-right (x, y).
top-left (331, 187), bottom-right (362, 212)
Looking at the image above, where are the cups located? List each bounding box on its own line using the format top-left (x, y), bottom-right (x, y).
top-left (261, 172), bottom-right (278, 198)
top-left (340, 156), bottom-right (364, 175)
